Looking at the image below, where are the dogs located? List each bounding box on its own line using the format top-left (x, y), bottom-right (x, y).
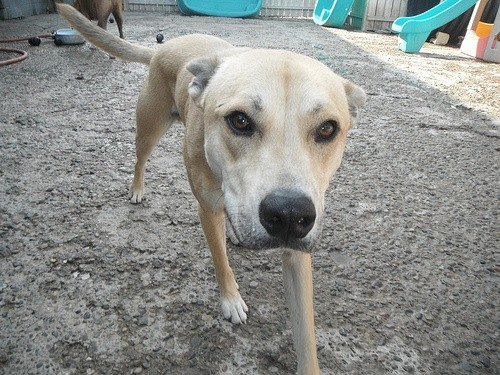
top-left (55, 3), bottom-right (367, 375)
top-left (69, 0), bottom-right (124, 39)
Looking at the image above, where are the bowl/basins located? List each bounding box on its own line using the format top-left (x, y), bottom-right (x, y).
top-left (55, 28), bottom-right (84, 44)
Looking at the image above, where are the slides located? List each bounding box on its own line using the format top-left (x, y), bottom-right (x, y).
top-left (391, 0), bottom-right (481, 35)
